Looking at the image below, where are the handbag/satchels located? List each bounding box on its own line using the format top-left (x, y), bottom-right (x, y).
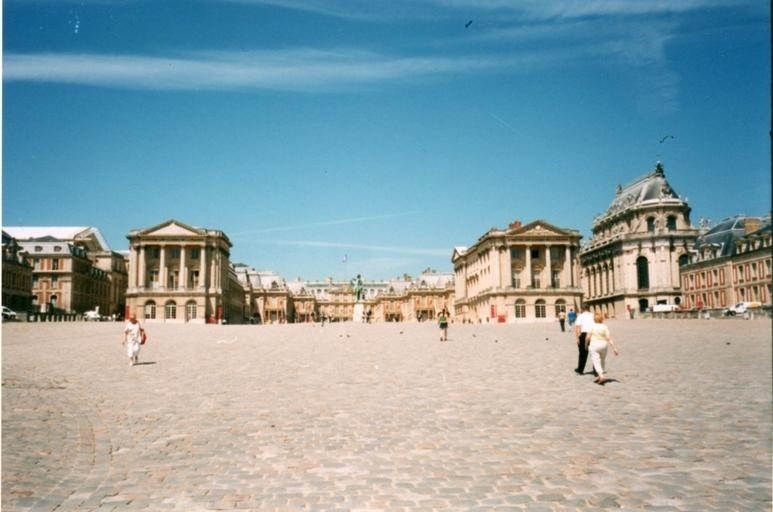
top-left (141, 329), bottom-right (147, 345)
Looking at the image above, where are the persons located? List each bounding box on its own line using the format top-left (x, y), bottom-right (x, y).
top-left (558, 308), bottom-right (566, 331)
top-left (568, 310), bottom-right (577, 332)
top-left (574, 303), bottom-right (598, 377)
top-left (122, 313), bottom-right (143, 368)
top-left (583, 313), bottom-right (619, 385)
top-left (436, 308), bottom-right (450, 343)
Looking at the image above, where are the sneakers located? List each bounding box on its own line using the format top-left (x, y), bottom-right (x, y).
top-left (575, 369), bottom-right (583, 374)
top-left (598, 378), bottom-right (606, 384)
